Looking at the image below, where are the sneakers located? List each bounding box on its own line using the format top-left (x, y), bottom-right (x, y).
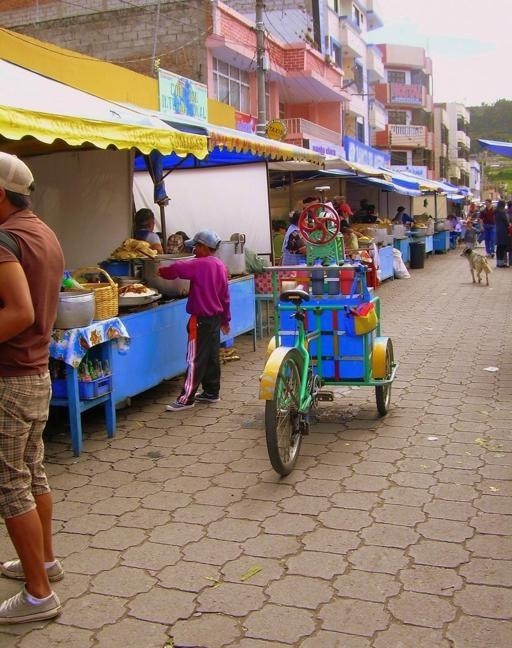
top-left (164, 400), bottom-right (194, 411)
top-left (3, 559), bottom-right (64, 581)
top-left (195, 392), bottom-right (220, 402)
top-left (0, 583), bottom-right (63, 623)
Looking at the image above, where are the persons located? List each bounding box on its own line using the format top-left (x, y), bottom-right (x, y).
top-left (272, 197), bottom-right (380, 266)
top-left (167, 233), bottom-right (175, 254)
top-left (156, 228), bottom-right (231, 411)
top-left (280, 208), bottom-right (311, 329)
top-left (393, 206), bottom-right (406, 224)
top-left (172, 231), bottom-right (190, 254)
top-left (0, 149), bottom-right (65, 622)
top-left (135, 207), bottom-right (166, 254)
top-left (445, 198), bottom-right (511, 267)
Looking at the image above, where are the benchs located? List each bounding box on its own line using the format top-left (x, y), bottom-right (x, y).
top-left (254, 267), bottom-right (297, 337)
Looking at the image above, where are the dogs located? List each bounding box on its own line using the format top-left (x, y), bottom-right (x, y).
top-left (460, 246), bottom-right (493, 286)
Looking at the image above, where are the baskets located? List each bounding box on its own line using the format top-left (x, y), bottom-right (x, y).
top-left (66, 267), bottom-right (119, 319)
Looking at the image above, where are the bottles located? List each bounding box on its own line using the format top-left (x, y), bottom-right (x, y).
top-left (312, 259), bottom-right (324, 295)
top-left (326, 260), bottom-right (340, 295)
top-left (339, 259), bottom-right (354, 294)
top-left (352, 259), bottom-right (365, 293)
top-left (295, 259), bottom-right (310, 294)
top-left (48, 356), bottom-right (111, 382)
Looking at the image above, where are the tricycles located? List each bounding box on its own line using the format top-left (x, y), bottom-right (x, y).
top-left (246, 258), bottom-right (394, 476)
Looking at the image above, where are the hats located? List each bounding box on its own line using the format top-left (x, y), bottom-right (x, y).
top-left (184, 229), bottom-right (221, 249)
top-left (1, 151), bottom-right (34, 195)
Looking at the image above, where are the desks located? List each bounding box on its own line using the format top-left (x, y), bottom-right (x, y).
top-left (43, 274), bottom-right (257, 456)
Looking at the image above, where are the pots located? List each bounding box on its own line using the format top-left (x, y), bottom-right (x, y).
top-left (141, 251), bottom-right (197, 296)
top-left (214, 232), bottom-right (247, 276)
